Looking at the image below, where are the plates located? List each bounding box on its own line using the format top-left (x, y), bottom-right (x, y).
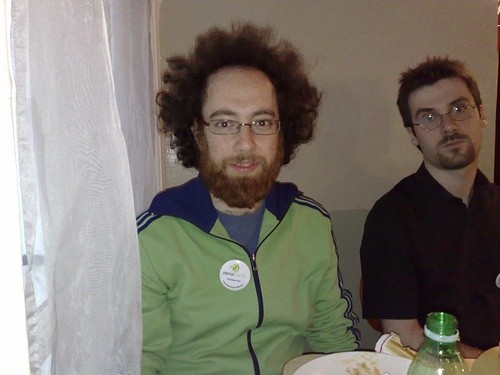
top-left (290, 351), bottom-right (434, 375)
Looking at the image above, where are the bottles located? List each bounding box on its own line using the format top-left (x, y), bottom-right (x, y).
top-left (406, 312), bottom-right (470, 375)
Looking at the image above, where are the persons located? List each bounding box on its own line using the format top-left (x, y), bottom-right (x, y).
top-left (357, 54), bottom-right (500, 359)
top-left (134, 20), bottom-right (361, 375)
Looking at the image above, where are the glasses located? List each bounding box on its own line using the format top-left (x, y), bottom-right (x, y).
top-left (203, 119), bottom-right (280, 135)
top-left (412, 103), bottom-right (477, 131)
